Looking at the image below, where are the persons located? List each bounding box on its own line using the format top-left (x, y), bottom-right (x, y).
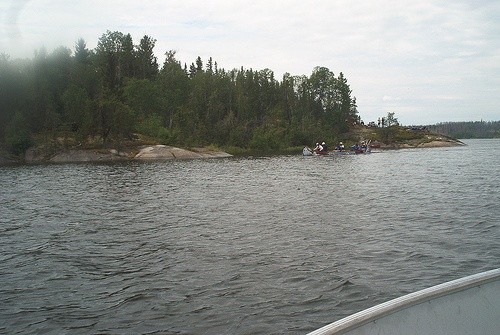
top-left (339, 141), bottom-right (346, 150)
top-left (321, 140), bottom-right (328, 151)
top-left (314, 142), bottom-right (323, 151)
top-left (353, 139), bottom-right (372, 151)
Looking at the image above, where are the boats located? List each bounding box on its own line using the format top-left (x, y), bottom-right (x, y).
top-left (355, 147), bottom-right (372, 154)
top-left (332, 149), bottom-right (355, 156)
top-left (303, 146), bottom-right (329, 157)
